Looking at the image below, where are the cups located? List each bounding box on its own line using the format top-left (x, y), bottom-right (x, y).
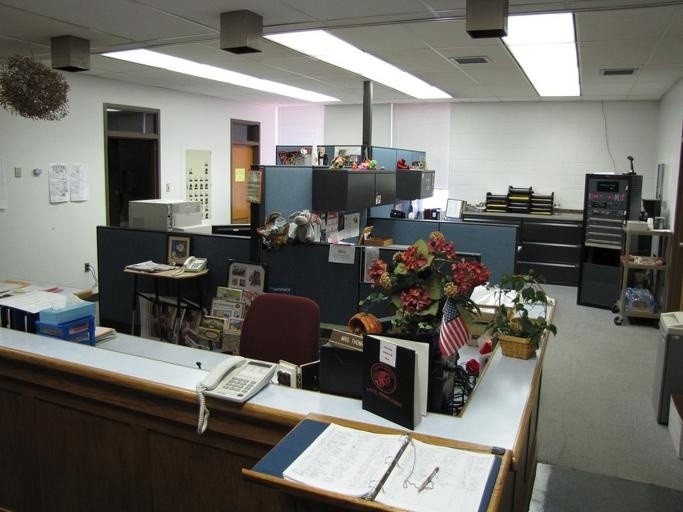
top-left (654, 217), bottom-right (664, 229)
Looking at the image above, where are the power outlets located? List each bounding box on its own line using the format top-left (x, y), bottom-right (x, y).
top-left (83, 262), bottom-right (89, 272)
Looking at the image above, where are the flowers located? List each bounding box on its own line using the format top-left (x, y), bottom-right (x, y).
top-left (358, 232), bottom-right (491, 338)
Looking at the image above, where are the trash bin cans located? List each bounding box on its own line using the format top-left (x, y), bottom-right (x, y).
top-left (652, 311), bottom-right (682, 425)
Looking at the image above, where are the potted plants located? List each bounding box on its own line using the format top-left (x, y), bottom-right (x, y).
top-left (485, 267), bottom-right (560, 359)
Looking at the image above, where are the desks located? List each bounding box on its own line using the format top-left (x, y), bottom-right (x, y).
top-left (96, 326), bottom-right (277, 381)
top-left (122, 264), bottom-right (210, 343)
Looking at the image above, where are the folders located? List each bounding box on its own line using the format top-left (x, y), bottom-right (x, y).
top-left (253, 420), bottom-right (502, 512)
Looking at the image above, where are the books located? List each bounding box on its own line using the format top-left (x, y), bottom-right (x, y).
top-left (196, 287), bottom-right (247, 355)
top-left (94, 325), bottom-right (116, 344)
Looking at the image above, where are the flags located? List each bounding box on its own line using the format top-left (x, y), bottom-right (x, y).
top-left (438, 295), bottom-right (472, 360)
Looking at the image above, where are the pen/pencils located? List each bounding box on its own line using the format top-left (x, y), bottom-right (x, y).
top-left (417, 466), bottom-right (440, 493)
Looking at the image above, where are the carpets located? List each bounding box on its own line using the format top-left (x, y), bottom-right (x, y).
top-left (505, 278), bottom-right (683, 495)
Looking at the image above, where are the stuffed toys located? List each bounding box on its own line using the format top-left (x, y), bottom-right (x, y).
top-left (256, 208), bottom-right (322, 251)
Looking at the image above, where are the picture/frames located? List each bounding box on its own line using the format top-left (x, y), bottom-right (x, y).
top-left (164, 235), bottom-right (190, 265)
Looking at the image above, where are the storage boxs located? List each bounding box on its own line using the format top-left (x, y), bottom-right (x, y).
top-left (362, 230), bottom-right (392, 247)
top-left (665, 393), bottom-right (682, 462)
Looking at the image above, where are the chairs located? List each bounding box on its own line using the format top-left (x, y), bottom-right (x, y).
top-left (235, 292), bottom-right (321, 370)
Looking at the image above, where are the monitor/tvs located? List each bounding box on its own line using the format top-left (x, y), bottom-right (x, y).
top-left (446, 198), bottom-right (467, 218)
top-left (390, 209), bottom-right (405, 218)
top-left (212, 224), bottom-right (251, 237)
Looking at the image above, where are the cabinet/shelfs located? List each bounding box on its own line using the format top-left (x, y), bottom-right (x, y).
top-left (34, 314), bottom-right (96, 347)
top-left (459, 207), bottom-right (583, 289)
top-left (311, 162), bottom-right (397, 215)
top-left (483, 185), bottom-right (555, 218)
top-left (394, 166), bottom-right (435, 201)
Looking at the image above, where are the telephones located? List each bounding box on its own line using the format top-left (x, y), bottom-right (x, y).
top-left (198, 356), bottom-right (277, 404)
top-left (183, 256), bottom-right (208, 272)
